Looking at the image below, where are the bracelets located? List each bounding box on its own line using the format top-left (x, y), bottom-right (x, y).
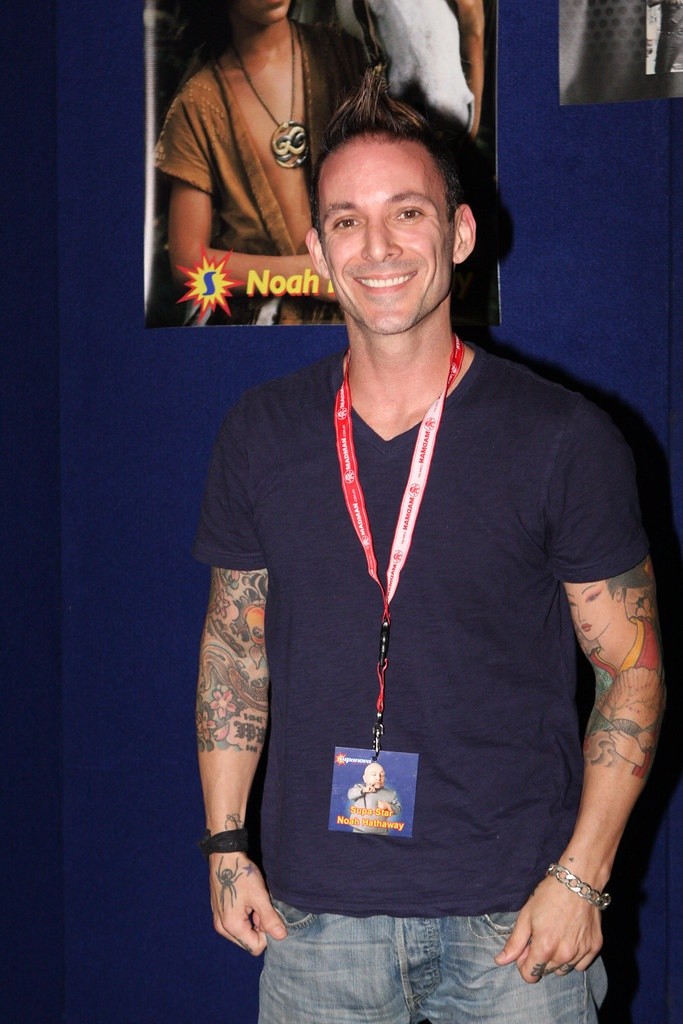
top-left (545, 861), bottom-right (611, 912)
top-left (198, 827), bottom-right (248, 860)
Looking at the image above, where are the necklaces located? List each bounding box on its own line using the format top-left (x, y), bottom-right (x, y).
top-left (229, 21), bottom-right (308, 167)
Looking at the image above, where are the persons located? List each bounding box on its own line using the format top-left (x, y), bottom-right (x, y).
top-left (156, 0), bottom-right (484, 325)
top-left (347, 764), bottom-right (402, 834)
top-left (193, 66), bottom-right (669, 1022)
